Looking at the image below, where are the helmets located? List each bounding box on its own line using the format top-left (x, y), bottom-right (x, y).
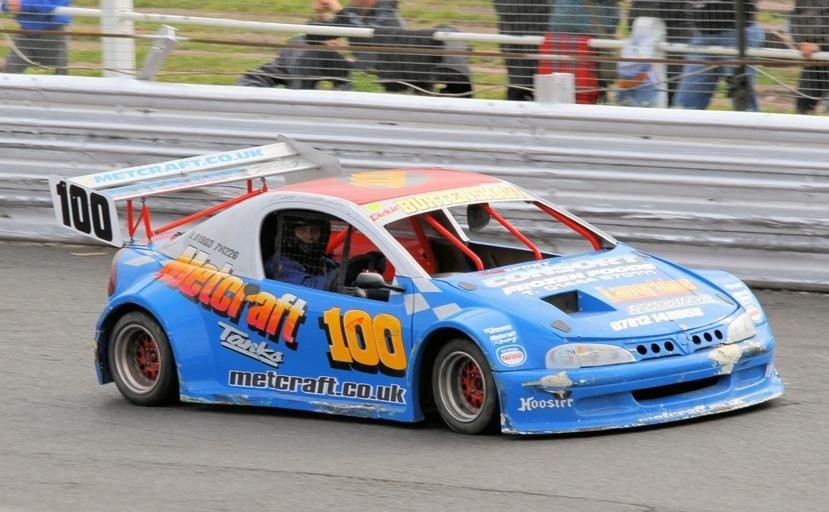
top-left (280, 210), bottom-right (331, 259)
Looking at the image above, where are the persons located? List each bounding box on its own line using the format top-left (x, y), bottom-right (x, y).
top-left (667, 0), bottom-right (769, 112)
top-left (1, 0), bottom-right (70, 75)
top-left (787, 0), bottom-right (828, 116)
top-left (302, 0), bottom-right (415, 94)
top-left (266, 208), bottom-right (387, 299)
top-left (489, 1), bottom-right (699, 110)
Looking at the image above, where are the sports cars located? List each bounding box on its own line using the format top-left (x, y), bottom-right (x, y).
top-left (39, 129), bottom-right (787, 430)
